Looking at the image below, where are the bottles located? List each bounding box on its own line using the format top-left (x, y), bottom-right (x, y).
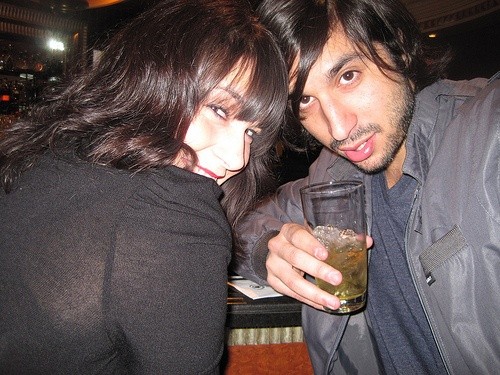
top-left (1, 40), bottom-right (60, 118)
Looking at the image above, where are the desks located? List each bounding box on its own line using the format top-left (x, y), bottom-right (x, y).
top-left (220, 269), bottom-right (317, 375)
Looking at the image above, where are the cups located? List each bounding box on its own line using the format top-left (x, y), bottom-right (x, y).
top-left (298, 178), bottom-right (368, 315)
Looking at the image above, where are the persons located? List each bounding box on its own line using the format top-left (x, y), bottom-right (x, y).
top-left (1, 0), bottom-right (290, 375)
top-left (217, 0), bottom-right (499, 374)
